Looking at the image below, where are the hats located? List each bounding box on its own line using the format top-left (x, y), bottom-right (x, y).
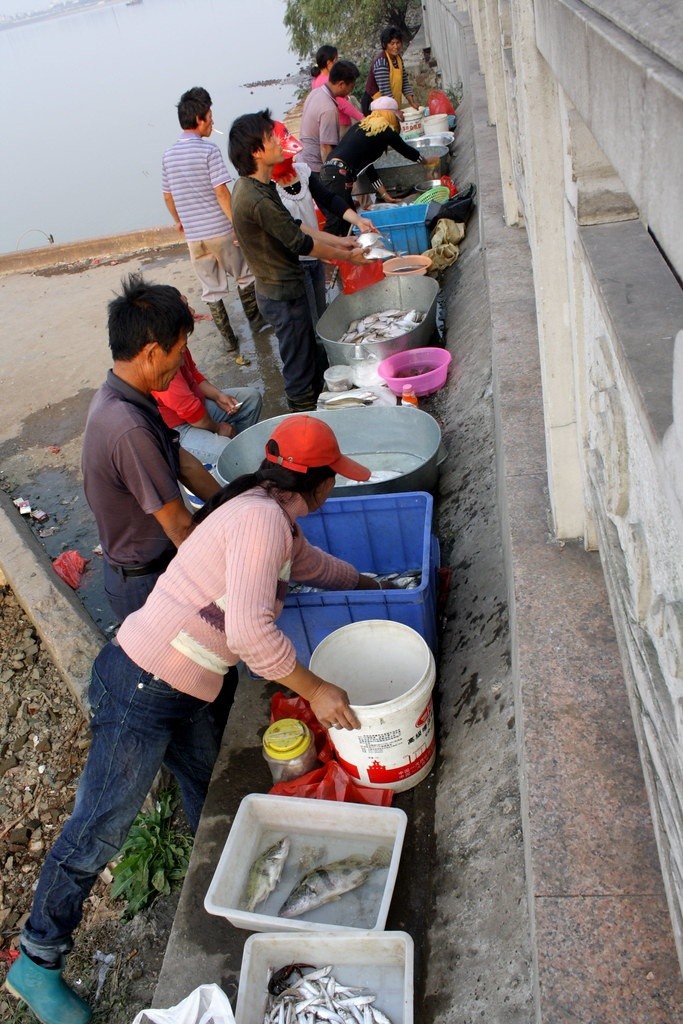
top-left (272, 122), bottom-right (301, 160)
top-left (265, 415), bottom-right (371, 482)
top-left (371, 97), bottom-right (399, 111)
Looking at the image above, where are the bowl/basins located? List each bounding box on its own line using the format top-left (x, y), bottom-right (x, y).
top-left (378, 347), bottom-right (451, 397)
top-left (402, 130), bottom-right (455, 145)
top-left (323, 364), bottom-right (353, 392)
top-left (415, 179), bottom-right (455, 192)
top-left (383, 254), bottom-right (432, 276)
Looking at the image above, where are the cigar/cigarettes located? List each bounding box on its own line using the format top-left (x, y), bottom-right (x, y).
top-left (211, 128), bottom-right (223, 135)
top-left (235, 403), bottom-right (242, 407)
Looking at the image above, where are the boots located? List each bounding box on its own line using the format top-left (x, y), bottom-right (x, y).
top-left (238, 282), bottom-right (268, 333)
top-left (5, 943), bottom-right (91, 1024)
top-left (209, 299), bottom-right (237, 351)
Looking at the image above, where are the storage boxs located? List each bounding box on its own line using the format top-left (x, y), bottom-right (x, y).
top-left (203, 793), bottom-right (407, 936)
top-left (246, 491), bottom-right (440, 681)
top-left (352, 204), bottom-right (432, 255)
top-left (233, 930), bottom-right (415, 1024)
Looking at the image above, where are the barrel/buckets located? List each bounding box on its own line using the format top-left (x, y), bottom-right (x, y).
top-left (307, 619), bottom-right (435, 793)
top-left (399, 105), bottom-right (425, 138)
top-left (422, 115), bottom-right (449, 134)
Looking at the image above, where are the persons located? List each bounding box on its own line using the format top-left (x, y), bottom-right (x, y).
top-left (300, 44), bottom-right (439, 236)
top-left (160, 87), bottom-right (265, 352)
top-left (148, 288), bottom-right (263, 486)
top-left (362, 27), bottom-right (420, 117)
top-left (5, 415), bottom-right (402, 1024)
top-left (270, 121), bottom-right (377, 353)
top-left (81, 273), bottom-right (238, 734)
top-left (228, 109), bottom-right (378, 413)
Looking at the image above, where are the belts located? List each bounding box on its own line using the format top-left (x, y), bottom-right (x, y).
top-left (320, 161), bottom-right (350, 173)
top-left (110, 555), bottom-right (161, 577)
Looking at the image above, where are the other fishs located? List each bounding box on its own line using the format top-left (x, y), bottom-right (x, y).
top-left (354, 231), bottom-right (383, 249)
top-left (278, 847), bottom-right (392, 918)
top-left (244, 837), bottom-right (290, 912)
top-left (263, 963), bottom-right (391, 1024)
top-left (364, 247), bottom-right (395, 260)
top-left (396, 367), bottom-right (434, 378)
top-left (337, 308), bottom-right (425, 344)
top-left (292, 572), bottom-right (420, 591)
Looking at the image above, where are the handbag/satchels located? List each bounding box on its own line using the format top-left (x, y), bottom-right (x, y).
top-left (428, 90), bottom-right (456, 117)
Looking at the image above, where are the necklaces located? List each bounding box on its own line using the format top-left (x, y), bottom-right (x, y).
top-left (258, 483), bottom-right (294, 530)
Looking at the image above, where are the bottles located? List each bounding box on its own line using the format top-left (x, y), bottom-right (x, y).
top-left (262, 718), bottom-right (316, 785)
top-left (402, 384), bottom-right (419, 411)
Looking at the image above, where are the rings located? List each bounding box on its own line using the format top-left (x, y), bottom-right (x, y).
top-left (333, 721), bottom-right (341, 728)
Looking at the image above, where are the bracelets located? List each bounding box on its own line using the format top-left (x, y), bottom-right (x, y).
top-left (376, 580), bottom-right (385, 591)
top-left (380, 192), bottom-right (387, 198)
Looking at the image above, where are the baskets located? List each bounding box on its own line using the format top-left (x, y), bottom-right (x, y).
top-left (415, 186), bottom-right (450, 205)
